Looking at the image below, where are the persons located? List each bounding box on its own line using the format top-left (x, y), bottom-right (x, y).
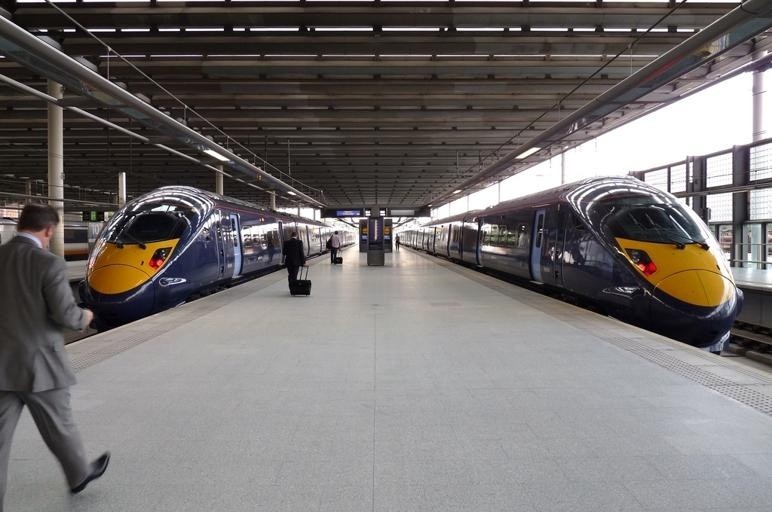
top-left (330, 231), bottom-right (341, 263)
top-left (395, 234), bottom-right (400, 250)
top-left (283, 231), bottom-right (306, 293)
top-left (0, 204), bottom-right (110, 512)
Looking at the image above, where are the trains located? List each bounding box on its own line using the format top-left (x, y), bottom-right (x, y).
top-left (394, 176), bottom-right (744, 355)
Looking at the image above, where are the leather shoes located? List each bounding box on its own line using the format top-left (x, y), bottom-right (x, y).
top-left (71, 451), bottom-right (110, 494)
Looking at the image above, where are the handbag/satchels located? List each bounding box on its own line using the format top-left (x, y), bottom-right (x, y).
top-left (326, 239), bottom-right (332, 249)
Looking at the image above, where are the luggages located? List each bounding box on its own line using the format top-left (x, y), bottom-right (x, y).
top-left (290, 279), bottom-right (312, 296)
top-left (334, 256), bottom-right (343, 265)
